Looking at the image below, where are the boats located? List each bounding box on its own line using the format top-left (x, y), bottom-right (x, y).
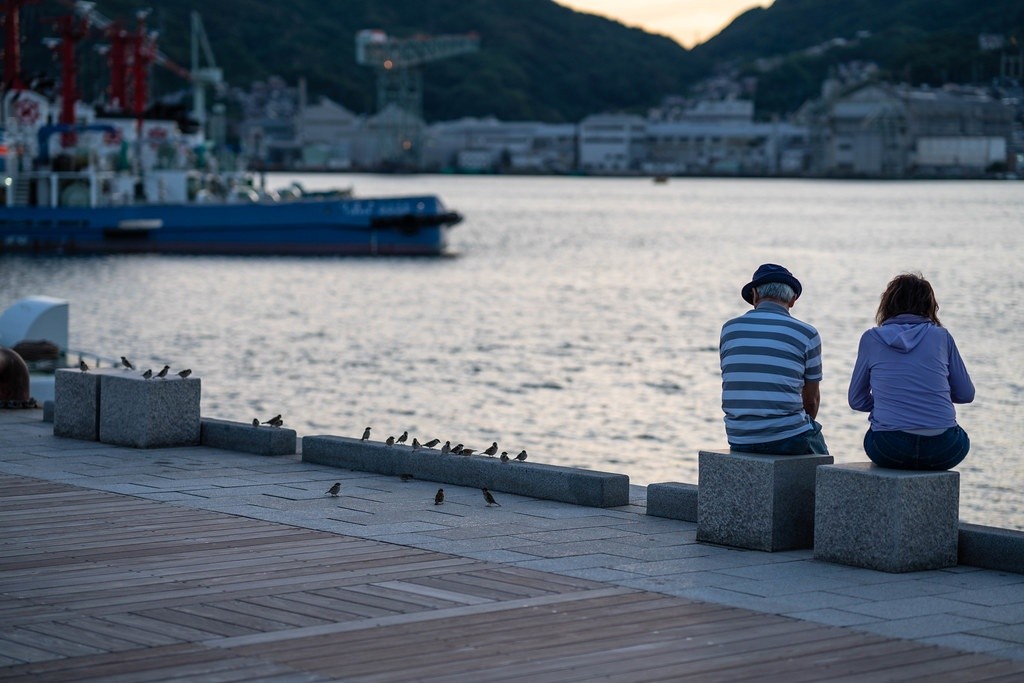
top-left (0, 89), bottom-right (465, 258)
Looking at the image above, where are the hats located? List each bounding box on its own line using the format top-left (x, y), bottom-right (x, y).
top-left (742, 264), bottom-right (802, 305)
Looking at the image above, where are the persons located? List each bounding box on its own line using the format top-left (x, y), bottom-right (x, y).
top-left (718, 264), bottom-right (831, 456)
top-left (847, 273), bottom-right (975, 471)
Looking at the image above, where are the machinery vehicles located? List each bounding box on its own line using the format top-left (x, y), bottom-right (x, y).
top-left (0, 0), bottom-right (191, 146)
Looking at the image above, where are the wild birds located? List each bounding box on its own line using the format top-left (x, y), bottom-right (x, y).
top-left (480, 442), bottom-right (498, 457)
top-left (140, 369), bottom-right (152, 380)
top-left (512, 450), bottom-right (528, 462)
top-left (395, 431), bottom-right (408, 444)
top-left (421, 438), bottom-right (441, 449)
top-left (325, 482), bottom-right (341, 496)
top-left (175, 369), bottom-right (192, 380)
top-left (384, 436), bottom-right (395, 446)
top-left (411, 438), bottom-right (422, 448)
top-left (80, 361), bottom-right (91, 374)
top-left (152, 366), bottom-right (170, 380)
top-left (450, 444), bottom-right (477, 456)
top-left (400, 473), bottom-right (413, 482)
top-left (261, 414), bottom-right (283, 428)
top-left (441, 440), bottom-right (451, 454)
top-left (499, 452), bottom-right (509, 463)
top-left (360, 426), bottom-right (372, 441)
top-left (252, 418), bottom-right (259, 427)
top-left (120, 356), bottom-right (135, 370)
top-left (435, 489), bottom-right (445, 504)
top-left (482, 488), bottom-right (501, 507)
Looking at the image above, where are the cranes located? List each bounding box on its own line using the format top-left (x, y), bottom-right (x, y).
top-left (188, 10), bottom-right (225, 137)
top-left (356, 27), bottom-right (483, 116)
